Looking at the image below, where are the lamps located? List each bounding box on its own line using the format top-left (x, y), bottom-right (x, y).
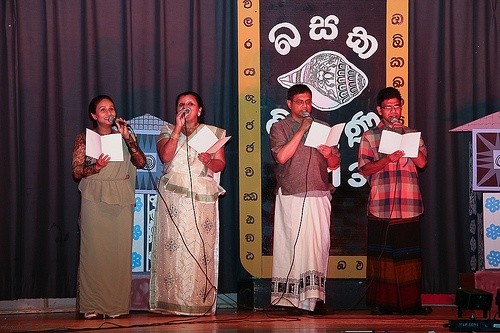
top-left (495, 288), bottom-right (500, 317)
top-left (455, 287), bottom-right (493, 319)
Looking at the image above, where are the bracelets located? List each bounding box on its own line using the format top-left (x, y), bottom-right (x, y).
top-left (171, 132), bottom-right (181, 142)
top-left (206, 160), bottom-right (211, 166)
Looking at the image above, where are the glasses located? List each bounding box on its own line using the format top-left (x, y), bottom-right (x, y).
top-left (291, 99), bottom-right (312, 104)
top-left (380, 104), bottom-right (401, 109)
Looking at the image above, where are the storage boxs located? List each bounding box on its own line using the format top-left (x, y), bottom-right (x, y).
top-left (235, 277), bottom-right (367, 315)
top-left (483, 193), bottom-right (500, 269)
top-left (474, 269), bottom-right (500, 313)
top-left (472, 128), bottom-right (500, 191)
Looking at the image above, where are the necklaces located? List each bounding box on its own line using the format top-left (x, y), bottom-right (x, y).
top-left (187, 123), bottom-right (199, 132)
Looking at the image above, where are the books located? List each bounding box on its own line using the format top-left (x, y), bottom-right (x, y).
top-left (188, 125), bottom-right (231, 155)
top-left (378, 130), bottom-right (421, 158)
top-left (85, 128), bottom-right (123, 161)
top-left (304, 121), bottom-right (346, 148)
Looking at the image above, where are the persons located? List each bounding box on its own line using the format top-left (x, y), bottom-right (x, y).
top-left (270, 84), bottom-right (341, 315)
top-left (149, 91), bottom-right (226, 316)
top-left (71, 95), bottom-right (146, 317)
top-left (358, 87), bottom-right (432, 315)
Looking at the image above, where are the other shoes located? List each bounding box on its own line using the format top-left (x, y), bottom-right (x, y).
top-left (85, 311), bottom-right (99, 319)
top-left (108, 314), bottom-right (121, 318)
top-left (315, 306), bottom-right (333, 314)
top-left (369, 305), bottom-right (385, 314)
top-left (406, 304), bottom-right (432, 315)
top-left (286, 306), bottom-right (302, 315)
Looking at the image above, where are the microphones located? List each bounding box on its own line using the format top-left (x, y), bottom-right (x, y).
top-left (391, 117), bottom-right (398, 123)
top-left (109, 116), bottom-right (130, 127)
top-left (302, 112), bottom-right (310, 134)
top-left (184, 108), bottom-right (191, 115)
